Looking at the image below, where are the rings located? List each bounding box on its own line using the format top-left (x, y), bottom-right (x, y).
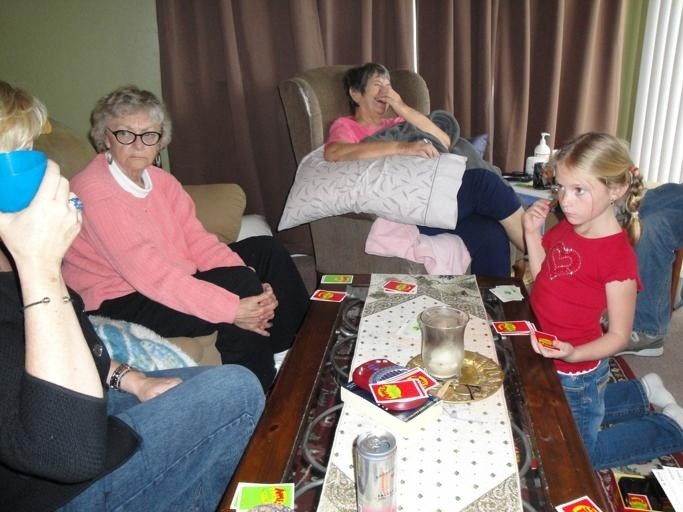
top-left (422, 138), bottom-right (433, 145)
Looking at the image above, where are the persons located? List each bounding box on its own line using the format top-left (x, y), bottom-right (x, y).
top-left (520, 131), bottom-right (683, 471)
top-left (0, 79), bottom-right (266, 512)
top-left (60, 82), bottom-right (312, 398)
top-left (323, 61), bottom-right (528, 279)
top-left (600, 182), bottom-right (683, 357)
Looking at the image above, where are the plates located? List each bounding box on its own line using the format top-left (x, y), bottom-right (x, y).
top-left (405, 350), bottom-right (506, 401)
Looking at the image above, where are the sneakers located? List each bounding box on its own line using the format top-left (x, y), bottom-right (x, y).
top-left (600, 310), bottom-right (663, 357)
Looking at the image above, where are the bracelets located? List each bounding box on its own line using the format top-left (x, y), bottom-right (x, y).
top-left (18, 296), bottom-right (73, 314)
top-left (118, 370), bottom-right (131, 392)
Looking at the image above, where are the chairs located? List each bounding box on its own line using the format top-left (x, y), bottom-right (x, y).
top-left (278, 62), bottom-right (498, 287)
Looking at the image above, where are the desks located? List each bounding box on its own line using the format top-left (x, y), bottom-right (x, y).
top-left (215, 276), bottom-right (612, 512)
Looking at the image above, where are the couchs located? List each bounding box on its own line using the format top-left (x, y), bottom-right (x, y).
top-left (1, 118), bottom-right (250, 370)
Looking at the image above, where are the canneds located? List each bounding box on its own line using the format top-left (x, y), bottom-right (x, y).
top-left (353, 429), bottom-right (398, 511)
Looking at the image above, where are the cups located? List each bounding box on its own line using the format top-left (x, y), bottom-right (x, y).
top-left (533, 162), bottom-right (554, 190)
top-left (417, 306), bottom-right (469, 380)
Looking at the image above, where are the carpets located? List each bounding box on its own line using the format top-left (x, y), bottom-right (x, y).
top-left (510, 268), bottom-right (682, 512)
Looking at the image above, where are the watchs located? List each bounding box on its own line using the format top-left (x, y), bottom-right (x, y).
top-left (110, 363), bottom-right (132, 390)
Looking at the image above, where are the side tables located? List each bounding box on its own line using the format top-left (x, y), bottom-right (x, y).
top-left (502, 174), bottom-right (560, 237)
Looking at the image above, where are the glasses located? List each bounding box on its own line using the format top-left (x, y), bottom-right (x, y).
top-left (106, 127), bottom-right (163, 147)
